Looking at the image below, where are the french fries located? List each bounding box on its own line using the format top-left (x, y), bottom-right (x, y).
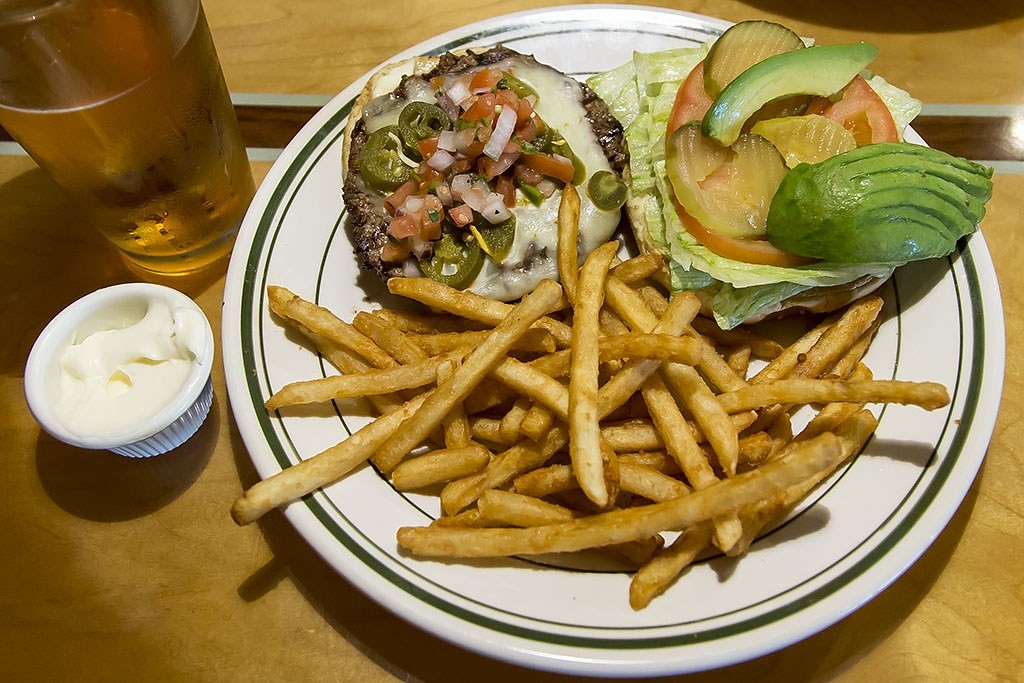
top-left (226, 185), bottom-right (951, 609)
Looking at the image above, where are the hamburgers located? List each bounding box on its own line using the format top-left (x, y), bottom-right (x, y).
top-left (341, 18), bottom-right (995, 326)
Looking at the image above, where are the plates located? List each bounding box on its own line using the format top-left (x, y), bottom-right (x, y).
top-left (222, 4), bottom-right (1004, 679)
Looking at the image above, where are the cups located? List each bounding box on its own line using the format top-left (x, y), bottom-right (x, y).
top-left (0, 0), bottom-right (256, 276)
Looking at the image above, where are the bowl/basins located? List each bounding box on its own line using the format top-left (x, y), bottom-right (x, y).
top-left (23, 283), bottom-right (214, 457)
